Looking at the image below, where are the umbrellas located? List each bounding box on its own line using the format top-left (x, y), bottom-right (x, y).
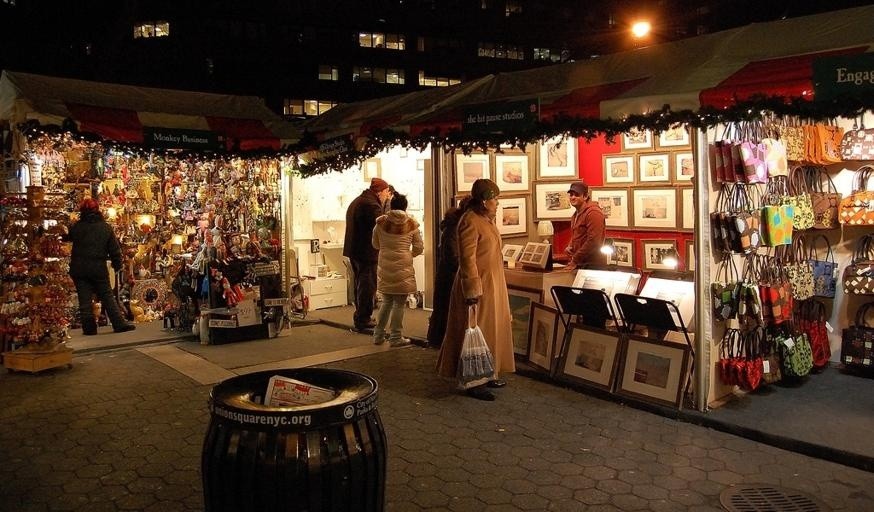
top-left (487, 378), bottom-right (506, 387)
top-left (459, 387), bottom-right (496, 401)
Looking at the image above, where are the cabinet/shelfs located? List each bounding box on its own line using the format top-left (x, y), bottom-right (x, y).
top-left (293, 276), bottom-right (348, 312)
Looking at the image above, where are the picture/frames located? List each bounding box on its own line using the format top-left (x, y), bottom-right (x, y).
top-left (588, 185), bottom-right (631, 230)
top-left (519, 242), bottom-right (553, 271)
top-left (493, 194), bottom-right (529, 238)
top-left (493, 148), bottom-right (532, 195)
top-left (685, 239), bottom-right (695, 274)
top-left (525, 301), bottom-right (560, 378)
top-left (637, 152), bottom-right (672, 186)
top-left (506, 282), bottom-right (545, 360)
top-left (654, 122), bottom-right (692, 152)
top-left (616, 334), bottom-right (689, 411)
top-left (640, 239), bottom-right (678, 273)
top-left (455, 195), bottom-right (469, 209)
top-left (557, 322), bottom-right (624, 393)
top-left (631, 186), bottom-right (679, 232)
top-left (672, 151), bottom-right (694, 187)
top-left (570, 263), bottom-right (643, 329)
top-left (679, 186), bottom-right (695, 233)
top-left (454, 150), bottom-right (493, 197)
top-left (602, 153), bottom-right (637, 186)
top-left (502, 244), bottom-right (523, 262)
top-left (620, 125), bottom-right (654, 153)
top-left (605, 237), bottom-right (637, 273)
top-left (532, 179), bottom-right (583, 222)
top-left (536, 131), bottom-right (579, 180)
top-left (636, 269), bottom-right (696, 332)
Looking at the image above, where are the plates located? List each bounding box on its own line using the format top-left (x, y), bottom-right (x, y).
top-left (293, 276), bottom-right (348, 312)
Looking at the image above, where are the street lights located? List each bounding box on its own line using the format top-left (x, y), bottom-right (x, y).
top-left (840, 110), bottom-right (874, 165)
top-left (838, 301), bottom-right (874, 374)
top-left (840, 232), bottom-right (874, 297)
top-left (711, 112), bottom-right (845, 392)
top-left (836, 163), bottom-right (874, 231)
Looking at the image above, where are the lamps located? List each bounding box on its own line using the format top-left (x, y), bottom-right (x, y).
top-left (537, 220), bottom-right (554, 244)
top-left (285, 248), bottom-right (308, 320)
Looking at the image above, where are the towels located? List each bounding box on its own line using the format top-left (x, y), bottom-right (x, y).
top-left (201, 366), bottom-right (390, 512)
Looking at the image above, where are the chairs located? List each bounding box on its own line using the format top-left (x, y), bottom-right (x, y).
top-left (355, 317), bottom-right (378, 329)
top-left (391, 337), bottom-right (412, 346)
top-left (83, 331), bottom-right (98, 335)
top-left (285, 248), bottom-right (308, 320)
top-left (114, 325), bottom-right (136, 332)
top-left (374, 337), bottom-right (385, 344)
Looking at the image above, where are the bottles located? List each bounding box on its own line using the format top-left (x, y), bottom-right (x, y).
top-left (567, 182), bottom-right (588, 195)
top-left (78, 198), bottom-right (99, 212)
top-left (370, 177), bottom-right (389, 193)
top-left (471, 179), bottom-right (499, 201)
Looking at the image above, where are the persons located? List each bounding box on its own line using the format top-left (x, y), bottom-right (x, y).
top-left (383, 184), bottom-right (395, 213)
top-left (435, 179), bottom-right (517, 402)
top-left (424, 193), bottom-right (473, 347)
top-left (371, 195), bottom-right (423, 346)
top-left (343, 178), bottom-right (390, 331)
top-left (60, 197), bottom-right (137, 334)
top-left (564, 180), bottom-right (608, 271)
